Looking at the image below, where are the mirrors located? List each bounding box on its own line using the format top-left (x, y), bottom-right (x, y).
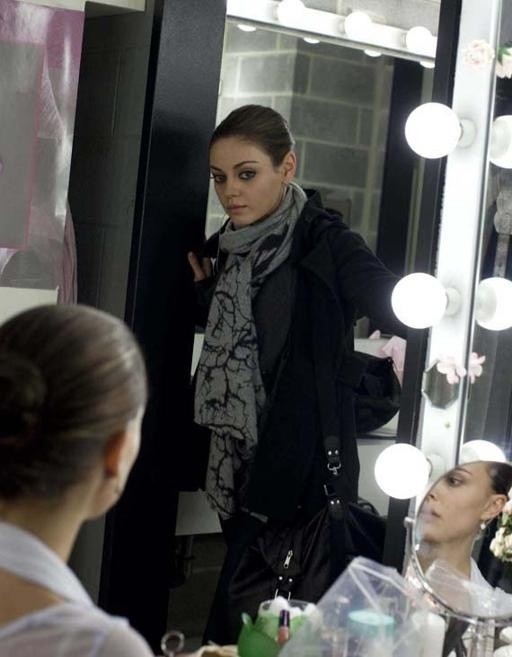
top-left (454, 0), bottom-right (512, 467)
top-left (412, 460), bottom-right (511, 657)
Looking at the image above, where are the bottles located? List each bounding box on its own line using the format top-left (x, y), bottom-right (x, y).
top-left (347, 611), bottom-right (394, 657)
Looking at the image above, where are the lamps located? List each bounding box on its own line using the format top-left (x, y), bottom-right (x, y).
top-left (239, 24), bottom-right (256, 32)
top-left (488, 114), bottom-right (512, 170)
top-left (475, 276), bottom-right (512, 331)
top-left (461, 440), bottom-right (508, 465)
top-left (374, 443), bottom-right (431, 499)
top-left (405, 25), bottom-right (431, 53)
top-left (304, 38), bottom-right (322, 44)
top-left (390, 273), bottom-right (459, 329)
top-left (277, 0), bottom-right (307, 24)
top-left (339, 13), bottom-right (373, 38)
top-left (420, 59), bottom-right (437, 72)
top-left (364, 47), bottom-right (382, 58)
top-left (174, 491), bottom-right (224, 558)
top-left (405, 102), bottom-right (478, 160)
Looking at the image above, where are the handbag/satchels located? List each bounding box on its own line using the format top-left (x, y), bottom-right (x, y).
top-left (275, 498), bottom-right (387, 603)
top-left (351, 348), bottom-right (405, 435)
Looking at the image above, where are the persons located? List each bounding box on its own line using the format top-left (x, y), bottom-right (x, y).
top-left (187, 104), bottom-right (405, 646)
top-left (415, 461), bottom-right (512, 590)
top-left (0, 302), bottom-right (154, 657)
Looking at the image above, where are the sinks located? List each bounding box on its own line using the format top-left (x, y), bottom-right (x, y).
top-left (354, 336), bottom-right (409, 438)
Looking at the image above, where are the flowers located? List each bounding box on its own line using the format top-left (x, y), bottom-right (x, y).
top-left (494, 46), bottom-right (512, 77)
top-left (468, 350), bottom-right (488, 387)
top-left (436, 356), bottom-right (468, 386)
